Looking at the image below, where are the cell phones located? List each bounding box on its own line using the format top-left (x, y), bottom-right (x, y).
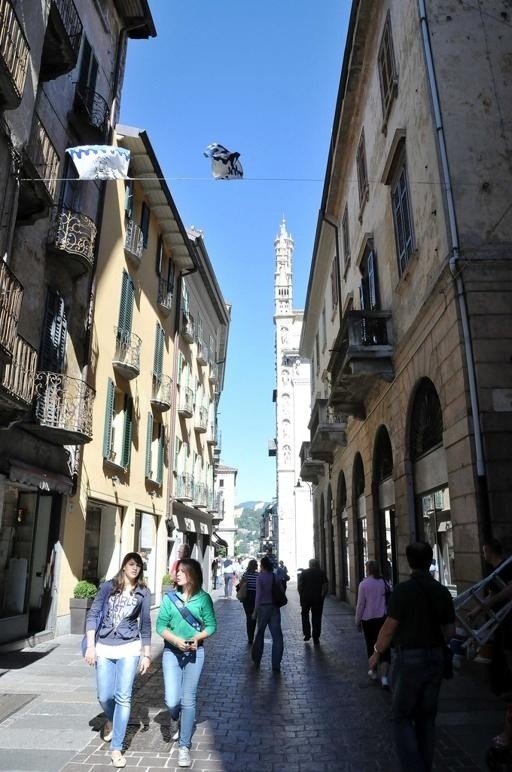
top-left (185, 641), bottom-right (194, 645)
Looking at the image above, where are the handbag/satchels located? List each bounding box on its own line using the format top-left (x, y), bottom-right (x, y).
top-left (271, 580), bottom-right (287, 606)
top-left (236, 582), bottom-right (248, 601)
top-left (82, 634), bottom-right (88, 657)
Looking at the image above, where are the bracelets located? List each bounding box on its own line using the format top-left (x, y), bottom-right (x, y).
top-left (144, 655), bottom-right (152, 661)
top-left (372, 643), bottom-right (384, 656)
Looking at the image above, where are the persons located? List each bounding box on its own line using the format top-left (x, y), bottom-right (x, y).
top-left (356, 557), bottom-right (392, 689)
top-left (367, 541), bottom-right (456, 772)
top-left (212, 555), bottom-right (287, 599)
top-left (84, 553), bottom-right (152, 768)
top-left (249, 557), bottom-right (284, 676)
top-left (298, 559), bottom-right (328, 646)
top-left (154, 558), bottom-right (217, 768)
top-left (481, 538), bottom-right (511, 703)
top-left (170, 543), bottom-right (190, 588)
top-left (237, 560), bottom-right (260, 647)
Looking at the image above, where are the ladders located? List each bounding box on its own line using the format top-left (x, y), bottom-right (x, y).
top-left (453, 555), bottom-right (512, 661)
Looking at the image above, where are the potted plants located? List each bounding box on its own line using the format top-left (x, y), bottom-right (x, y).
top-left (162, 575), bottom-right (175, 600)
top-left (70, 580), bottom-right (98, 634)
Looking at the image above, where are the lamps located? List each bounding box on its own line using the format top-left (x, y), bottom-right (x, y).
top-left (294, 479), bottom-right (314, 501)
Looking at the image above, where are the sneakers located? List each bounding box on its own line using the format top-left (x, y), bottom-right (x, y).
top-left (381, 677), bottom-right (389, 688)
top-left (314, 640), bottom-right (319, 644)
top-left (178, 747), bottom-right (191, 766)
top-left (303, 635), bottom-right (309, 641)
top-left (112, 753), bottom-right (126, 768)
top-left (368, 669), bottom-right (378, 680)
top-left (104, 720), bottom-right (113, 741)
top-left (170, 716), bottom-right (180, 740)
